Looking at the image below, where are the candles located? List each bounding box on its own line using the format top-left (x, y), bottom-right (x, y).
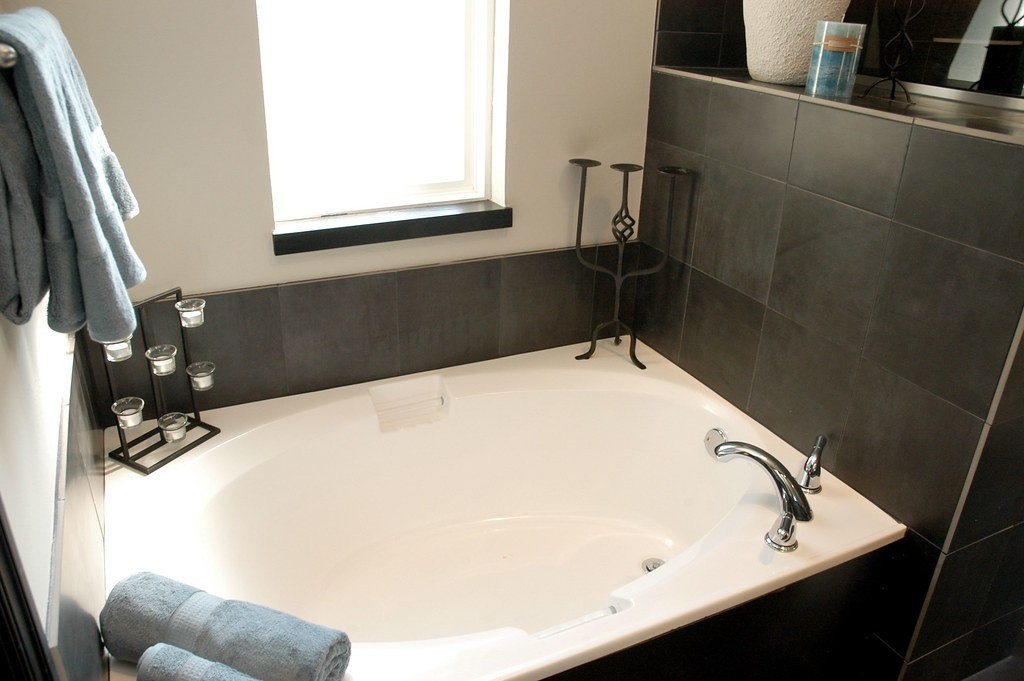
top-left (803, 14), bottom-right (866, 100)
top-left (102, 335), bottom-right (133, 363)
top-left (186, 360), bottom-right (216, 392)
top-left (111, 396), bottom-right (145, 428)
top-left (145, 344), bottom-right (177, 377)
top-left (175, 298), bottom-right (206, 329)
top-left (158, 412), bottom-right (188, 444)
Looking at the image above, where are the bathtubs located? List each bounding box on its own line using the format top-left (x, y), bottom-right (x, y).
top-left (105, 329), bottom-right (904, 679)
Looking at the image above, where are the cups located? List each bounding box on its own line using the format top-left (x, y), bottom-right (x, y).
top-left (158, 412), bottom-right (188, 443)
top-left (145, 345), bottom-right (178, 377)
top-left (185, 361), bottom-right (216, 391)
top-left (174, 299), bottom-right (206, 328)
top-left (110, 396), bottom-right (145, 429)
top-left (102, 332), bottom-right (133, 363)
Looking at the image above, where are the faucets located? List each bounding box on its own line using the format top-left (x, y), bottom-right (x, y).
top-left (713, 441), bottom-right (814, 522)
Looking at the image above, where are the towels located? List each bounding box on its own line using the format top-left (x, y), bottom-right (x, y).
top-left (0, 7), bottom-right (147, 341)
top-left (95, 570), bottom-right (351, 681)
